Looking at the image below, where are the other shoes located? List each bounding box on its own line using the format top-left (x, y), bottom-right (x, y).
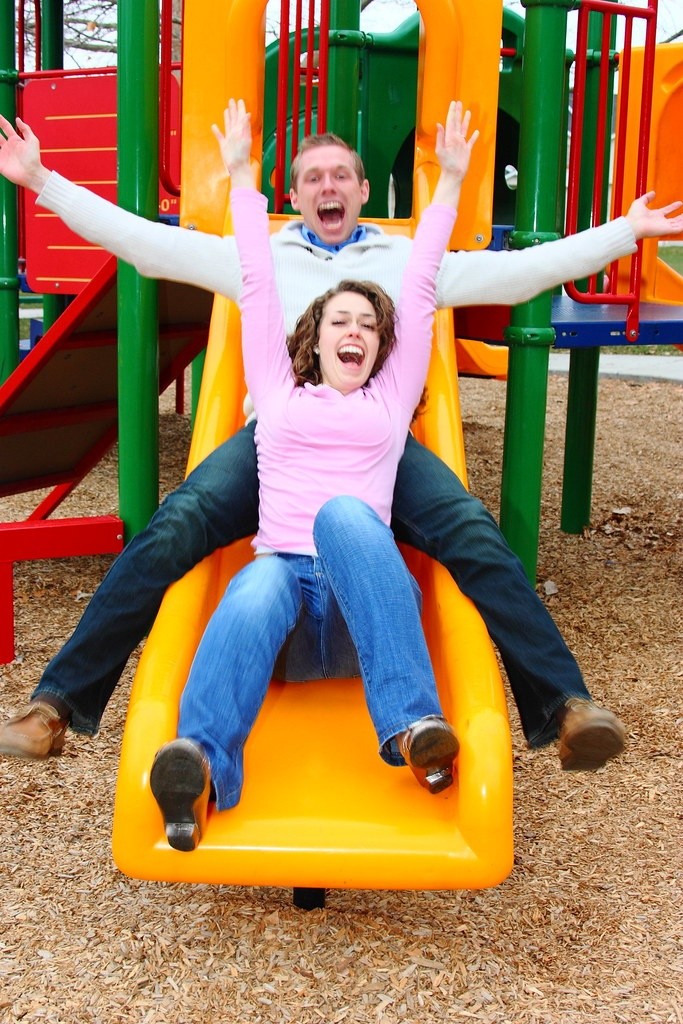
top-left (150, 736), bottom-right (211, 852)
top-left (558, 697), bottom-right (626, 770)
top-left (0, 700), bottom-right (70, 760)
top-left (395, 719), bottom-right (460, 794)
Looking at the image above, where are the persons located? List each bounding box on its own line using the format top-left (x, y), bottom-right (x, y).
top-left (0, 115), bottom-right (683, 772)
top-left (149, 94), bottom-right (479, 852)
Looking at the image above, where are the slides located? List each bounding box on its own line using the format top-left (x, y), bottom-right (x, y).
top-left (110, 201), bottom-right (516, 891)
